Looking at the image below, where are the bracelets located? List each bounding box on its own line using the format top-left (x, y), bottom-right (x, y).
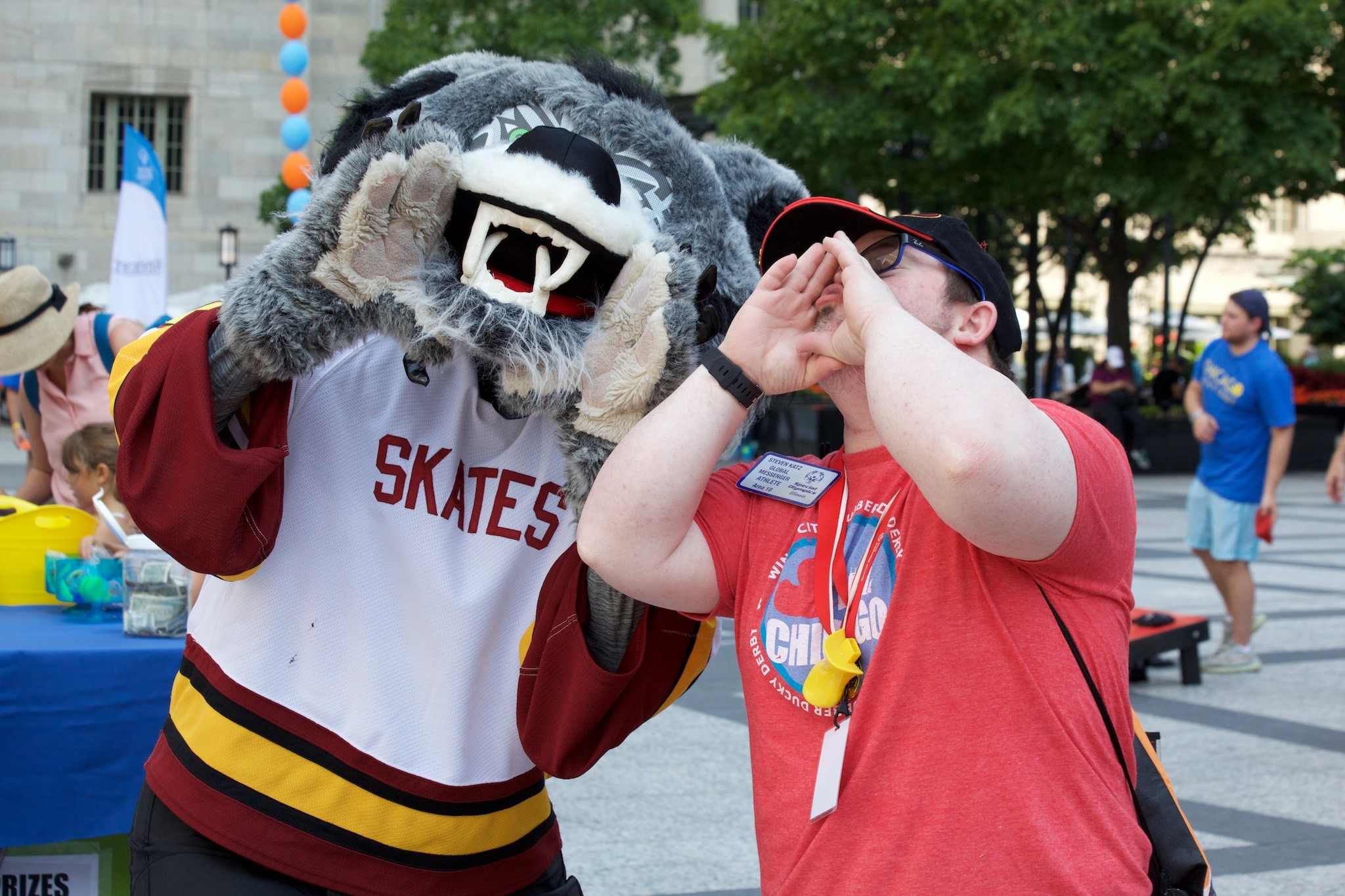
top-left (11, 422), bottom-right (22, 429)
top-left (1188, 409), bottom-right (1204, 424)
top-left (695, 346), bottom-right (762, 413)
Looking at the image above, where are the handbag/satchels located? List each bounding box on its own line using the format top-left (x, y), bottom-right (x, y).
top-left (1129, 709), bottom-right (1218, 896)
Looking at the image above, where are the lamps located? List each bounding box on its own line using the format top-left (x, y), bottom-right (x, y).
top-left (218, 224), bottom-right (237, 267)
top-left (0, 233), bottom-right (18, 271)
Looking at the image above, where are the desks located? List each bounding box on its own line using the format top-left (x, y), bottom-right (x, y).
top-left (0, 603), bottom-right (189, 845)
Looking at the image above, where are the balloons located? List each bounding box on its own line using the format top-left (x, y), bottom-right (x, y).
top-left (275, 3), bottom-right (316, 221)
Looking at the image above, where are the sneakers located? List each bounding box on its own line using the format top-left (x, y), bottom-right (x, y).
top-left (1198, 644), bottom-right (1261, 673)
top-left (1214, 613), bottom-right (1267, 656)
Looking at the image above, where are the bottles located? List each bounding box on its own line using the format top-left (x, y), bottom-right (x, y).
top-left (121, 535), bottom-right (192, 638)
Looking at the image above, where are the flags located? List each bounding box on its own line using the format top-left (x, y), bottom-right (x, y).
top-left (108, 123), bottom-right (167, 329)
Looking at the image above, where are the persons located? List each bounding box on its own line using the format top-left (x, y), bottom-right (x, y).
top-left (1181, 290), bottom-right (1295, 672)
top-left (1326, 430), bottom-right (1345, 505)
top-left (64, 421), bottom-right (142, 562)
top-left (1, 264), bottom-right (149, 510)
top-left (575, 191), bottom-right (1154, 896)
top-left (1297, 336), bottom-right (1322, 372)
top-left (1, 373), bottom-right (30, 451)
top-left (1035, 340), bottom-right (1196, 469)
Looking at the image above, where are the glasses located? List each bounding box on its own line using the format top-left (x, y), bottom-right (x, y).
top-left (859, 232), bottom-right (986, 301)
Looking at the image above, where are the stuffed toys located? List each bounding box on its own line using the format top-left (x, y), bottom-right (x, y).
top-left (106, 53), bottom-right (811, 896)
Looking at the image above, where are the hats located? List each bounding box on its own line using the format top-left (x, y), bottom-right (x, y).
top-left (0, 264), bottom-right (80, 377)
top-left (1232, 289), bottom-right (1272, 329)
top-left (759, 196), bottom-right (1022, 360)
top-left (1106, 346), bottom-right (1124, 368)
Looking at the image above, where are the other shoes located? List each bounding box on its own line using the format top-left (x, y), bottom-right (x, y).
top-left (1131, 450), bottom-right (1149, 468)
